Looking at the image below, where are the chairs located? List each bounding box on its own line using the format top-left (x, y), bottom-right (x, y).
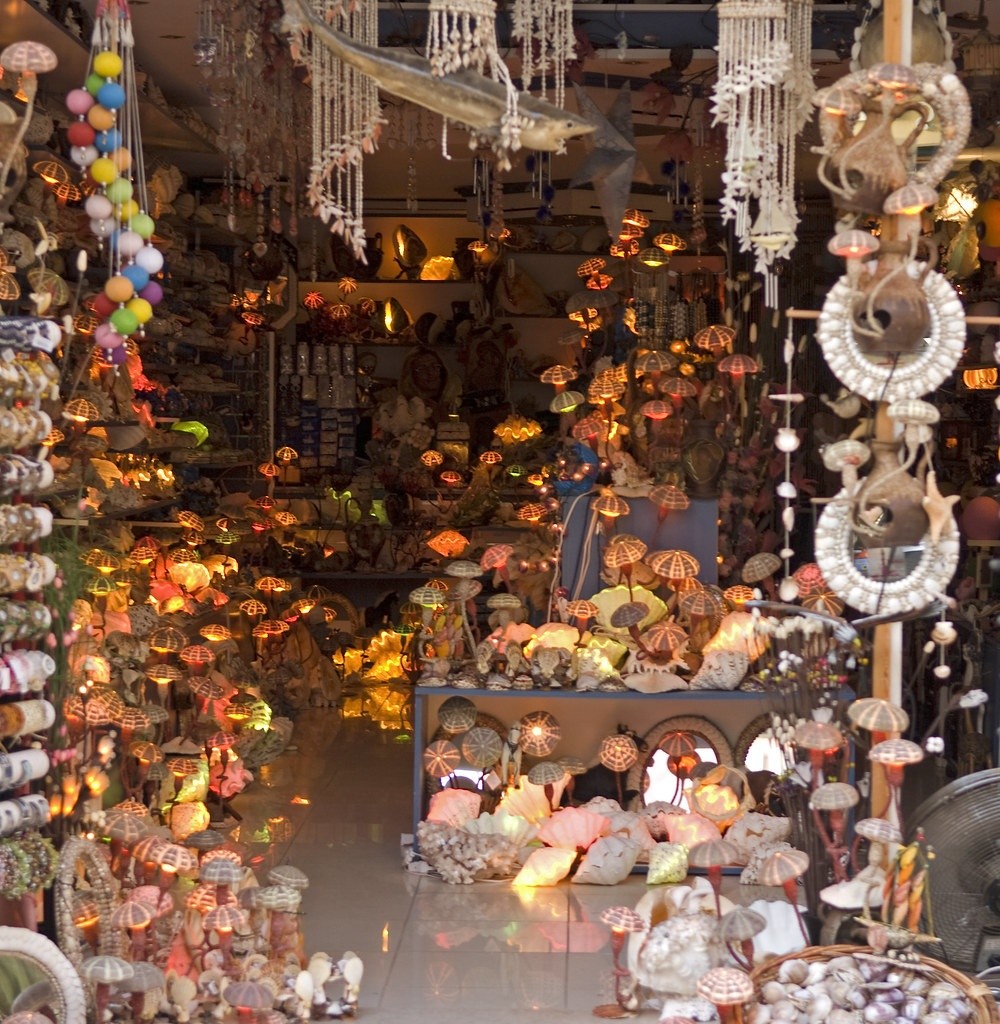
top-left (561, 496), bottom-right (720, 602)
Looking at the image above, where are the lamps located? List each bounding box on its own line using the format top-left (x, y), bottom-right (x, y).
top-left (34, 162), bottom-right (1000, 1024)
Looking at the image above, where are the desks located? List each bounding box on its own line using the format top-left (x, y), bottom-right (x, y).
top-left (413, 684), bottom-right (856, 875)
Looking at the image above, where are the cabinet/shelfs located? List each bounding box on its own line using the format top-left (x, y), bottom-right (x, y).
top-left (497, 205), bottom-right (738, 586)
top-left (276, 210), bottom-right (490, 581)
top-left (136, 211), bottom-right (274, 504)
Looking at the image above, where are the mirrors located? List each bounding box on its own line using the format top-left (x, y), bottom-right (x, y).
top-left (425, 713), bottom-right (509, 801)
top-left (734, 712), bottom-right (801, 776)
top-left (626, 715), bottom-right (735, 816)
